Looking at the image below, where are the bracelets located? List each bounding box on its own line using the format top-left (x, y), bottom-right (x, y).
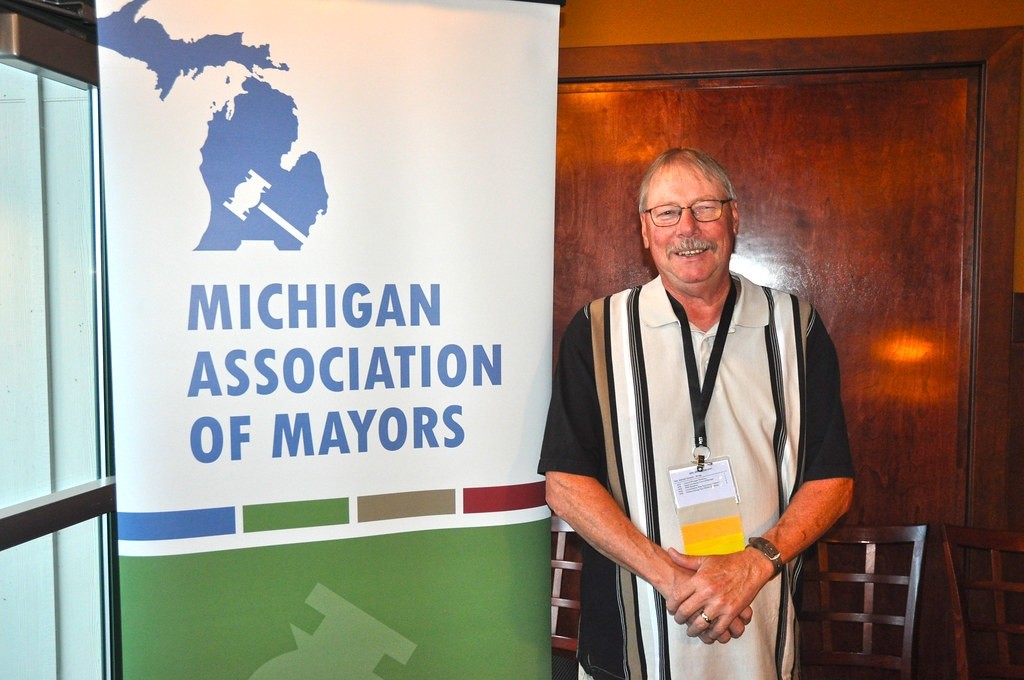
top-left (744, 535), bottom-right (782, 578)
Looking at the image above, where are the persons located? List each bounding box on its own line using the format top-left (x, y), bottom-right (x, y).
top-left (538, 144), bottom-right (853, 679)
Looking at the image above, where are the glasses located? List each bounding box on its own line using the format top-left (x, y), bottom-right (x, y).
top-left (644, 198), bottom-right (733, 228)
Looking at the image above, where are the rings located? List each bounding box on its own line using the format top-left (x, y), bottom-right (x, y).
top-left (700, 611), bottom-right (713, 624)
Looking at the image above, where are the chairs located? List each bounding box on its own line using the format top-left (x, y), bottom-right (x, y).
top-left (939, 521), bottom-right (1024, 680)
top-left (798, 524), bottom-right (930, 680)
top-left (551, 515), bottom-right (585, 680)
